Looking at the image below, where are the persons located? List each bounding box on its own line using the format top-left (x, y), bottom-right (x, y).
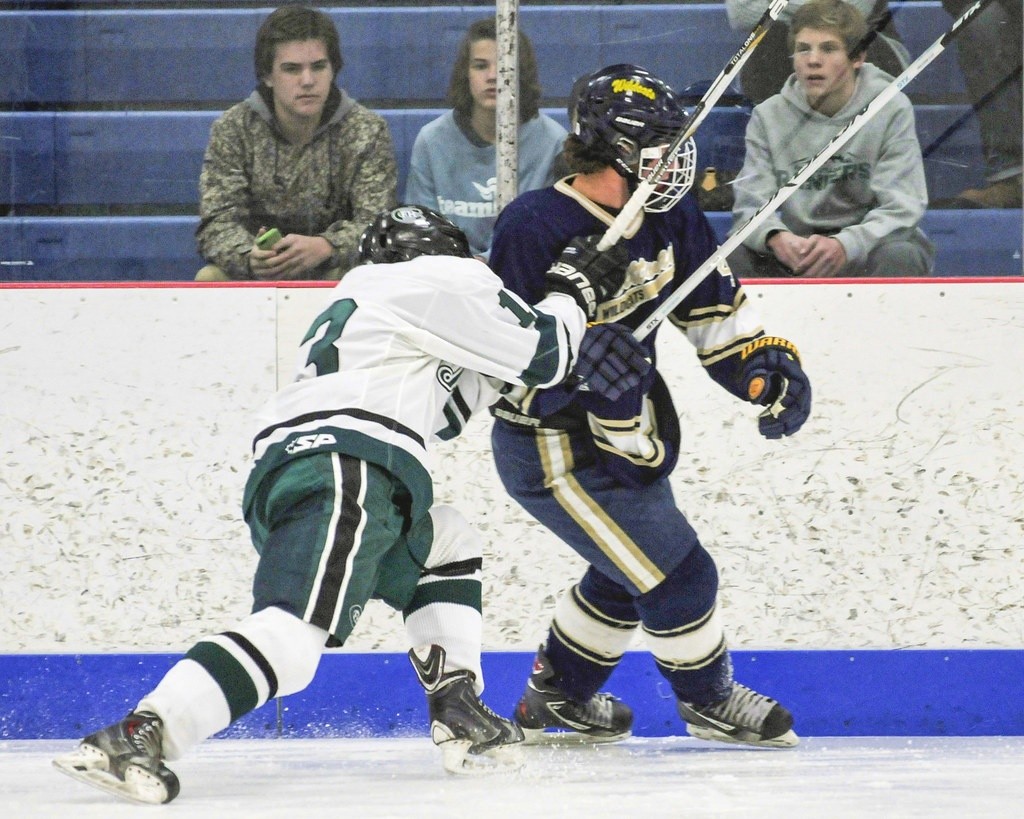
top-left (193, 5), bottom-right (398, 283)
top-left (721, 0), bottom-right (935, 278)
top-left (489, 62), bottom-right (811, 747)
top-left (404, 14), bottom-right (571, 262)
top-left (939, 0), bottom-right (1024, 211)
top-left (51, 204), bottom-right (633, 805)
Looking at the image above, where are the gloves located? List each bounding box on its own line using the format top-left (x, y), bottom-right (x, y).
top-left (543, 234), bottom-right (629, 322)
top-left (565, 320), bottom-right (651, 402)
top-left (737, 336), bottom-right (811, 439)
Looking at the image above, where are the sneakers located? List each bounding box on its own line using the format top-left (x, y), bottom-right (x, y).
top-left (51, 705), bottom-right (180, 806)
top-left (514, 643), bottom-right (633, 744)
top-left (407, 644), bottom-right (527, 776)
top-left (677, 680), bottom-right (799, 748)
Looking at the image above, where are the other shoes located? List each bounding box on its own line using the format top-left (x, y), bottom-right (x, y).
top-left (948, 173), bottom-right (1024, 209)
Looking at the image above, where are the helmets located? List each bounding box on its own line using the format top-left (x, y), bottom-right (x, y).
top-left (566, 62), bottom-right (697, 213)
top-left (358, 204), bottom-right (474, 266)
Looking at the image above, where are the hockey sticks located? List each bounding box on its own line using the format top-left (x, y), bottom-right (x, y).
top-left (597, 0), bottom-right (788, 251)
top-left (630, 1), bottom-right (994, 347)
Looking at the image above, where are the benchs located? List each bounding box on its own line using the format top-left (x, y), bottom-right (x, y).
top-left (0, 0), bottom-right (1024, 282)
